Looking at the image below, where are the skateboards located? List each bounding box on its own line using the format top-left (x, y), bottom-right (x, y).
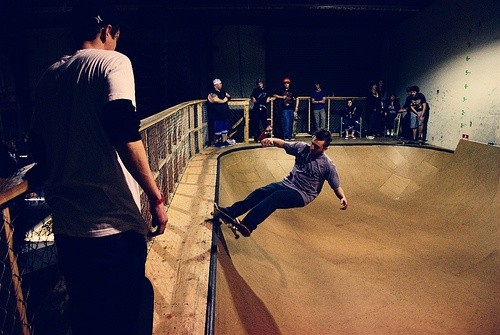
top-left (210, 202), bottom-right (251, 239)
top-left (258, 125), bottom-right (273, 140)
top-left (398, 140), bottom-right (427, 145)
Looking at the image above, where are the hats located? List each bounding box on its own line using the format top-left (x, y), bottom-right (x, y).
top-left (283, 78), bottom-right (291, 82)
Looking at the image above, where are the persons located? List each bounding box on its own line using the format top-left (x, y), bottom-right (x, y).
top-left (340, 98), bottom-right (361, 140)
top-left (250, 78), bottom-right (272, 143)
top-left (365, 81), bottom-right (382, 139)
top-left (25, 6), bottom-right (169, 334)
top-left (397, 86), bottom-right (412, 140)
top-left (207, 78), bottom-right (232, 148)
top-left (377, 79), bottom-right (388, 137)
top-left (310, 79), bottom-right (326, 130)
top-left (214, 127), bottom-right (348, 237)
top-left (384, 92), bottom-right (401, 136)
top-left (406, 85), bottom-right (427, 142)
top-left (273, 77), bottom-right (299, 140)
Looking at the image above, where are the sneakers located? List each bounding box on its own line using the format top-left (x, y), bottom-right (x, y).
top-left (235, 218), bottom-right (252, 233)
top-left (223, 140), bottom-right (232, 147)
top-left (214, 141), bottom-right (225, 148)
top-left (218, 205), bottom-right (235, 219)
